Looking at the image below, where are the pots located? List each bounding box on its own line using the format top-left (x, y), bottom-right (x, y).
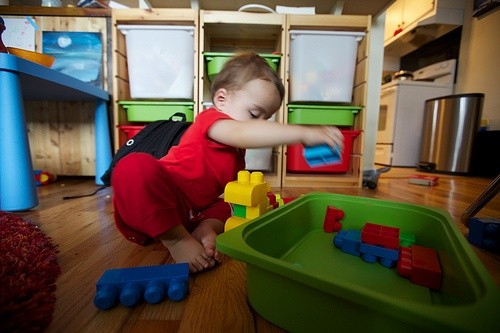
top-left (394, 70), bottom-right (414, 80)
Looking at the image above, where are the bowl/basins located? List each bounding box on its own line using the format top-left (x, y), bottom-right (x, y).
top-left (8, 47), bottom-right (55, 68)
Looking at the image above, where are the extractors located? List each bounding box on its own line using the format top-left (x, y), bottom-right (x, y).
top-left (384, 9), bottom-right (466, 57)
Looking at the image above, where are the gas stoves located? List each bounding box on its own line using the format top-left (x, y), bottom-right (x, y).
top-left (381, 80), bottom-right (449, 90)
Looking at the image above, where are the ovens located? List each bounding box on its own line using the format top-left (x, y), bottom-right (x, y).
top-left (375, 85), bottom-right (452, 167)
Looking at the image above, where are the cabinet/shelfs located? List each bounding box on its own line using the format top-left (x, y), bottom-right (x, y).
top-left (384, 0), bottom-right (464, 57)
top-left (111, 8), bottom-right (372, 190)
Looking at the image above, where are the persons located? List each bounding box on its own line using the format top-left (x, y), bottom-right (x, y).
top-left (111, 52), bottom-right (344, 272)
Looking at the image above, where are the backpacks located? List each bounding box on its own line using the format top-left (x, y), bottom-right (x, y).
top-left (61, 112), bottom-right (194, 202)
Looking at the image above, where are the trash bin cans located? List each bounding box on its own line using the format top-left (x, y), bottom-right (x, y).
top-left (416, 91), bottom-right (485, 176)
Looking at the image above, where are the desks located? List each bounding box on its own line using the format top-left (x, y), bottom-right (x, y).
top-left (0, 52), bottom-right (113, 212)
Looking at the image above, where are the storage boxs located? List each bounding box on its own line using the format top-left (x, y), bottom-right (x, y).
top-left (215, 191), bottom-right (500, 333)
top-left (116, 24), bottom-right (366, 174)
